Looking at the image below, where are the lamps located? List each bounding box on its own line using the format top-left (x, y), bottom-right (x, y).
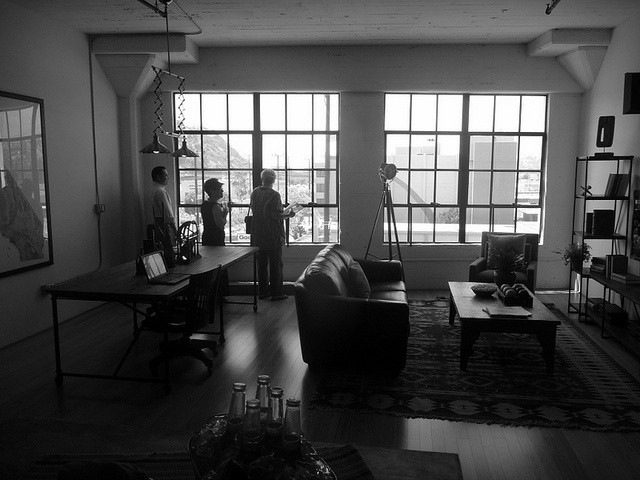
top-left (138, 0), bottom-right (199, 158)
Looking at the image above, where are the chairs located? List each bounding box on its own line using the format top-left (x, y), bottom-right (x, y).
top-left (155, 264), bottom-right (222, 377)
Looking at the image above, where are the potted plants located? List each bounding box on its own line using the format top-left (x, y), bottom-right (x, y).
top-left (554, 240), bottom-right (591, 269)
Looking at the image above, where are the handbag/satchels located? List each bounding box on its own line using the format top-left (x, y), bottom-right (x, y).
top-left (244, 216), bottom-right (253, 235)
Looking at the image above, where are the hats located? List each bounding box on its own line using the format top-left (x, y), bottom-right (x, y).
top-left (201, 177), bottom-right (224, 188)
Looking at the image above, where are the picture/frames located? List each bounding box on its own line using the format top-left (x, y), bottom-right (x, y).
top-left (0, 91), bottom-right (54, 278)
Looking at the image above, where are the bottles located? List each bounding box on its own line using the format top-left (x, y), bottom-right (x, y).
top-left (281, 399), bottom-right (302, 458)
top-left (240, 399), bottom-right (263, 458)
top-left (255, 374), bottom-right (271, 430)
top-left (266, 386), bottom-right (284, 450)
top-left (226, 382), bottom-right (245, 437)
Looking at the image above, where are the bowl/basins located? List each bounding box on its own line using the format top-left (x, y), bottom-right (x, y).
top-left (471, 285), bottom-right (496, 296)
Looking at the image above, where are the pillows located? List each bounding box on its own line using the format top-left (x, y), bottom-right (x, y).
top-left (486, 235), bottom-right (527, 270)
top-left (350, 259), bottom-right (370, 298)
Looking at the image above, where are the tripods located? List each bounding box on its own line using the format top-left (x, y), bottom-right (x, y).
top-left (364, 184), bottom-right (406, 281)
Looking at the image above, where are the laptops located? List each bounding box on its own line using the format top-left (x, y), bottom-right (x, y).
top-left (138, 254), bottom-right (191, 286)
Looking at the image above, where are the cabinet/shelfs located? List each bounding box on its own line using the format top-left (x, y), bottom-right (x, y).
top-left (568, 153), bottom-right (633, 322)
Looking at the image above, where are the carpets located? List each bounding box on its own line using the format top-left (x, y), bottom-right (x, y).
top-left (0, 418), bottom-right (464, 480)
top-left (308, 298), bottom-right (640, 433)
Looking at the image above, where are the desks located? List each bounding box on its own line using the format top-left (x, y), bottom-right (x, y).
top-left (584, 273), bottom-right (640, 360)
top-left (48, 245), bottom-right (261, 393)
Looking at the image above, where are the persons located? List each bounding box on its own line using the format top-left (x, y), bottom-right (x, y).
top-left (200, 178), bottom-right (230, 303)
top-left (246, 170), bottom-right (294, 302)
top-left (147, 167), bottom-right (177, 250)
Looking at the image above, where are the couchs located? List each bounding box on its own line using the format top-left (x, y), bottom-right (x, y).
top-left (295, 241), bottom-right (410, 369)
top-left (468, 231), bottom-right (539, 296)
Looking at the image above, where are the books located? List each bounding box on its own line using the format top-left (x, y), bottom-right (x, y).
top-left (613, 272), bottom-right (639, 284)
top-left (604, 173), bottom-right (630, 197)
top-left (585, 209), bottom-right (616, 236)
top-left (590, 257), bottom-right (608, 275)
top-left (483, 306), bottom-right (531, 317)
top-left (585, 298), bottom-right (629, 327)
top-left (606, 254), bottom-right (629, 280)
